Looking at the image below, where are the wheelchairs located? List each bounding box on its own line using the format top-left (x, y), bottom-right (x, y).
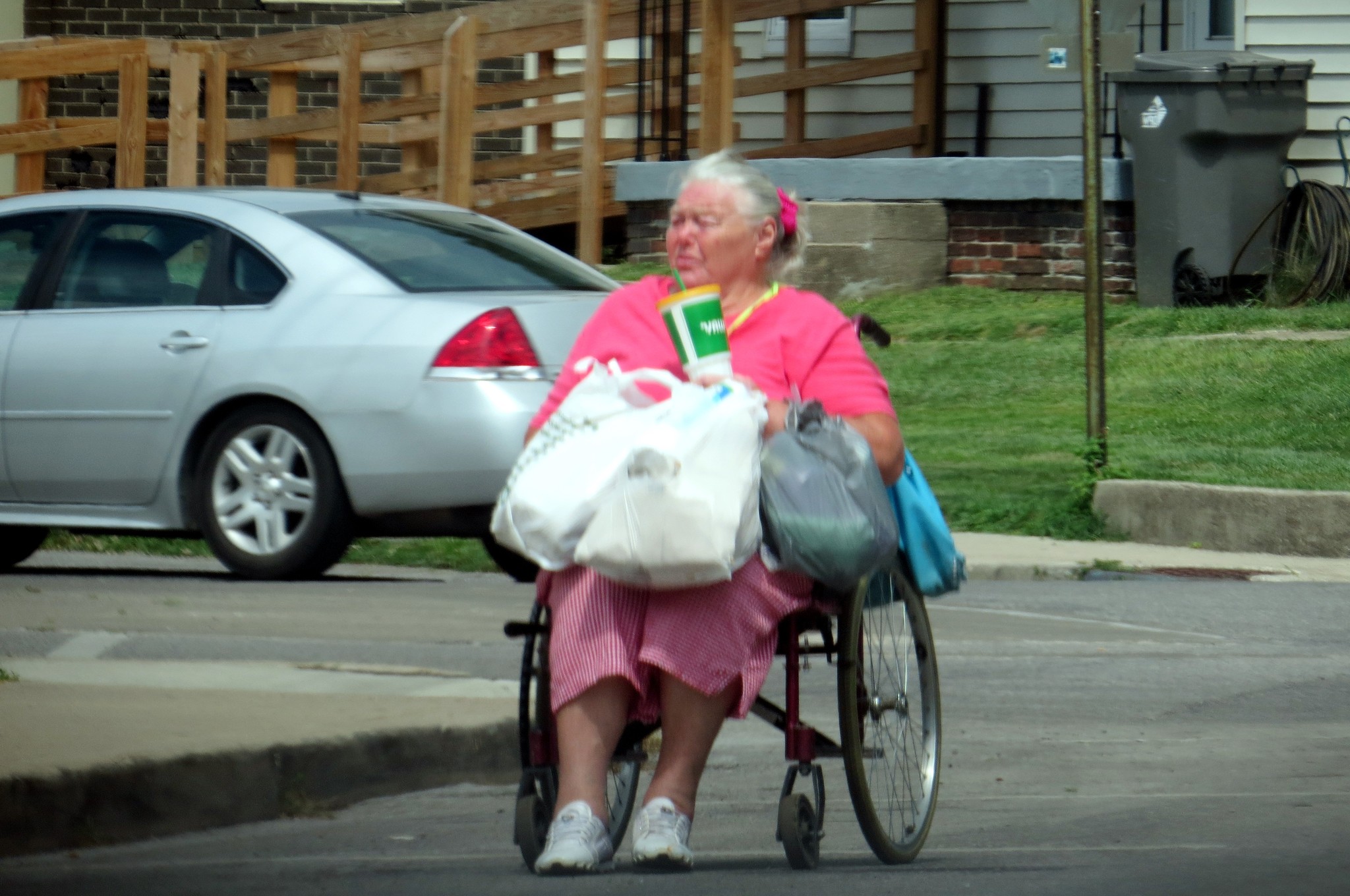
top-left (503, 310), bottom-right (945, 870)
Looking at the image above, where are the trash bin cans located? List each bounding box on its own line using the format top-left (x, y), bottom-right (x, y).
top-left (1109, 50), bottom-right (1315, 314)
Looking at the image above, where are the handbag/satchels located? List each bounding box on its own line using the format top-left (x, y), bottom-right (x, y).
top-left (851, 447), bottom-right (965, 608)
top-left (572, 375), bottom-right (763, 591)
top-left (759, 390), bottom-right (900, 589)
top-left (489, 369), bottom-right (704, 574)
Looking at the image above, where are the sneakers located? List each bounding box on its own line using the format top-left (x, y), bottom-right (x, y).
top-left (533, 799), bottom-right (615, 875)
top-left (631, 797), bottom-right (695, 871)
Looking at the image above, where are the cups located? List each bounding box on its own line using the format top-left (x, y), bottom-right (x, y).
top-left (655, 283), bottom-right (734, 383)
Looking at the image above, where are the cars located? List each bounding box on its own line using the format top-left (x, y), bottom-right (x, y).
top-left (0, 187), bottom-right (627, 583)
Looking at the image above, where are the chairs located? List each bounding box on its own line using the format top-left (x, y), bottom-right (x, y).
top-left (81, 237), bottom-right (197, 306)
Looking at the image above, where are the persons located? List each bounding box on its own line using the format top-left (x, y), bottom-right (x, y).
top-left (523, 147), bottom-right (905, 873)
